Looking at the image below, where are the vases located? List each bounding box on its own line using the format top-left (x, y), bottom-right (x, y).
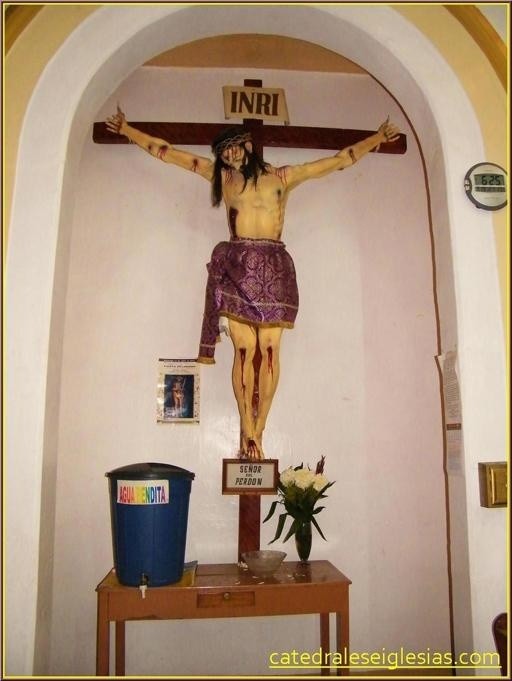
top-left (295, 520), bottom-right (312, 562)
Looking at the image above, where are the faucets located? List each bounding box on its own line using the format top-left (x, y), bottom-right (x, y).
top-left (136, 572), bottom-right (150, 599)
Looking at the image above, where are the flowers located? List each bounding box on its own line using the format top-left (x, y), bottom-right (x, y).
top-left (262, 455), bottom-right (338, 545)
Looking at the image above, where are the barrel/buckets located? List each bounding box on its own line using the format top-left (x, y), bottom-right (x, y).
top-left (106, 464), bottom-right (195, 585)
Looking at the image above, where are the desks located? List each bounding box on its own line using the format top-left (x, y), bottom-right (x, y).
top-left (95, 560), bottom-right (352, 676)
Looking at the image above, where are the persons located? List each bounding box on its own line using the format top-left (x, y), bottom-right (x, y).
top-left (105, 106), bottom-right (399, 460)
top-left (171, 375), bottom-right (184, 413)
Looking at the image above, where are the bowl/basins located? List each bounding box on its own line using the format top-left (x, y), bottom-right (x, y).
top-left (242, 551), bottom-right (287, 580)
